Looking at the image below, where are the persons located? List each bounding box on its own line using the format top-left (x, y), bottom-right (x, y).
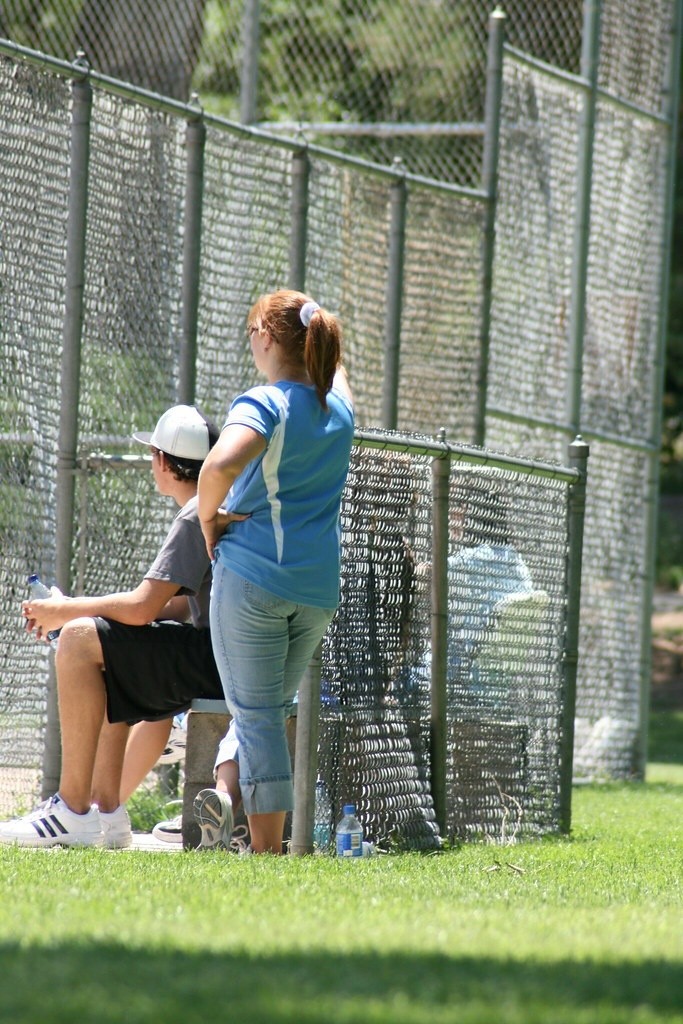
top-left (151, 451), bottom-right (534, 843)
top-left (0, 404), bottom-right (227, 850)
top-left (191, 290), bottom-right (355, 855)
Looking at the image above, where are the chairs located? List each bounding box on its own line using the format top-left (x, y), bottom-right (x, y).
top-left (459, 591), bottom-right (551, 712)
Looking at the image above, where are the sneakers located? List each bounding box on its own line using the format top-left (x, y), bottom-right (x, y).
top-left (100, 804), bottom-right (132, 850)
top-left (193, 788), bottom-right (234, 854)
top-left (0, 795), bottom-right (103, 848)
top-left (153, 814), bottom-right (184, 843)
top-left (156, 723), bottom-right (186, 763)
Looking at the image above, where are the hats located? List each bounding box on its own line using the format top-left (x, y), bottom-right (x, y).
top-left (132, 404), bottom-right (220, 461)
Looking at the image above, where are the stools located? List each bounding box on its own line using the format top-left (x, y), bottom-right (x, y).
top-left (182, 698), bottom-right (299, 855)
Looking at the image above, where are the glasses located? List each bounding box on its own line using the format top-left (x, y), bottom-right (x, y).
top-left (247, 323), bottom-right (280, 345)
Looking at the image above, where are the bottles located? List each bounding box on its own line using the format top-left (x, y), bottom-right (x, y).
top-left (336, 806), bottom-right (364, 856)
top-left (28, 574), bottom-right (63, 650)
top-left (314, 780), bottom-right (332, 848)
